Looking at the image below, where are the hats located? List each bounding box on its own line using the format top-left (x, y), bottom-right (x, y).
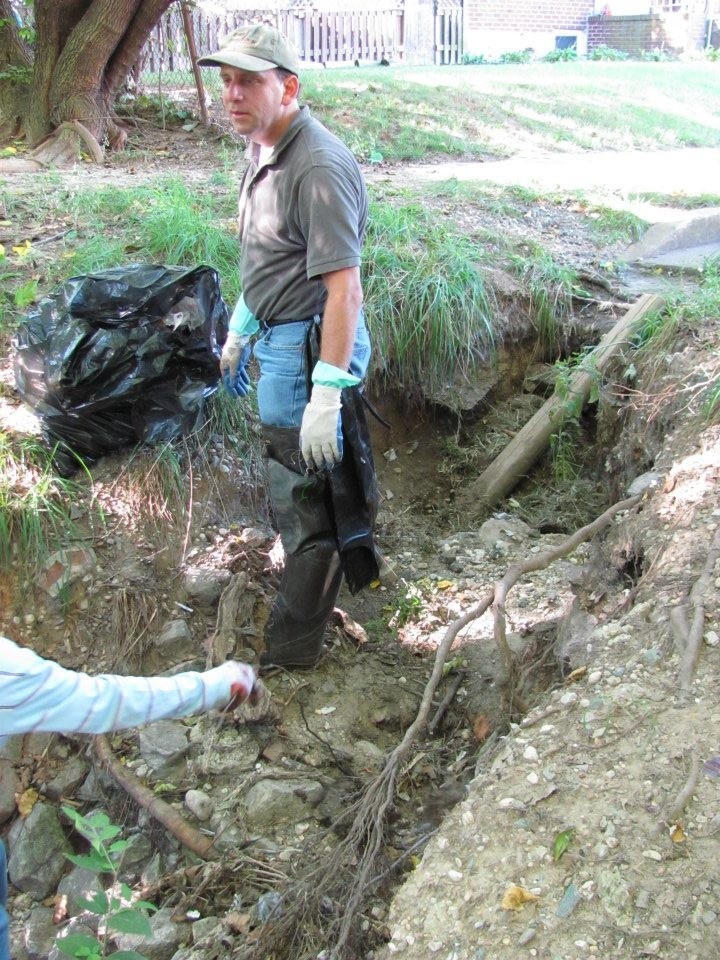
top-left (197, 21), bottom-right (300, 75)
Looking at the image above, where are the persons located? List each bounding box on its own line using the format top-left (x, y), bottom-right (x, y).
top-left (0, 638), bottom-right (258, 960)
top-left (196, 24), bottom-right (381, 680)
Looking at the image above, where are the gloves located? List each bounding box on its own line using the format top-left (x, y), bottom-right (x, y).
top-left (299, 358), bottom-right (360, 469)
top-left (203, 664), bottom-right (255, 712)
top-left (220, 292), bottom-right (260, 398)
